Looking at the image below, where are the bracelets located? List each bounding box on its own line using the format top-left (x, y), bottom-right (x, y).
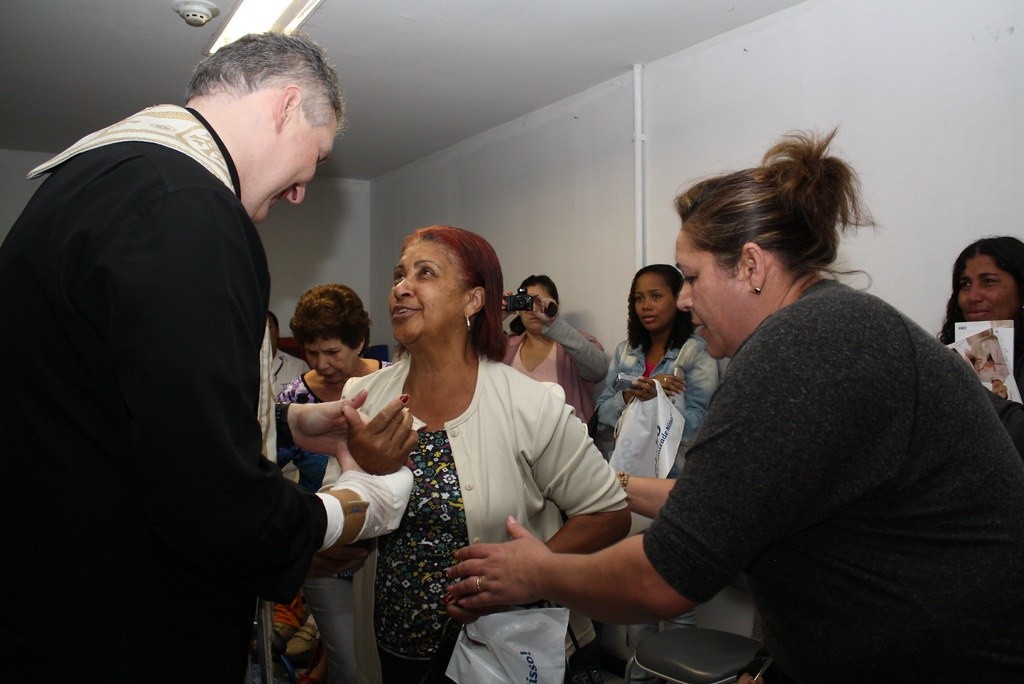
top-left (275, 401), bottom-right (294, 449)
top-left (619, 472), bottom-right (628, 491)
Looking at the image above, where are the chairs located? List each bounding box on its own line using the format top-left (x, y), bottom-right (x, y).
top-left (624, 601), bottom-right (774, 684)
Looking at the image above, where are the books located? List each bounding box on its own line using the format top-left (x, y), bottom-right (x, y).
top-left (946, 319), bottom-right (1023, 404)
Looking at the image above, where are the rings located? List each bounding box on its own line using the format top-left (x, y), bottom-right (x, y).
top-left (477, 575), bottom-right (484, 591)
top-left (664, 378), bottom-right (666, 382)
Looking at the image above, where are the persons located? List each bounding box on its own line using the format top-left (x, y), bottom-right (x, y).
top-left (939, 236), bottom-right (1024, 460)
top-left (0, 31), bottom-right (414, 684)
top-left (446, 128), bottom-right (1024, 684)
top-left (276, 284), bottom-right (393, 684)
top-left (502, 274), bottom-right (608, 424)
top-left (964, 328), bottom-right (1010, 400)
top-left (323, 225), bottom-right (633, 684)
top-left (593, 264), bottom-right (720, 479)
top-left (267, 310), bottom-right (310, 382)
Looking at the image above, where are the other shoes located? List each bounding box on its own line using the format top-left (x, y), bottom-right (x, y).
top-left (252, 622), bottom-right (297, 650)
top-left (284, 622), bottom-right (320, 658)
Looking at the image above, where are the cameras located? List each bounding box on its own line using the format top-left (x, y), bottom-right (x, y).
top-left (503, 287), bottom-right (533, 312)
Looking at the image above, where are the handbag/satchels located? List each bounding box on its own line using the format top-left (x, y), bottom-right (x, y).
top-left (609, 378), bottom-right (685, 537)
top-left (570, 667), bottom-right (603, 684)
top-left (445, 607), bottom-right (570, 684)
